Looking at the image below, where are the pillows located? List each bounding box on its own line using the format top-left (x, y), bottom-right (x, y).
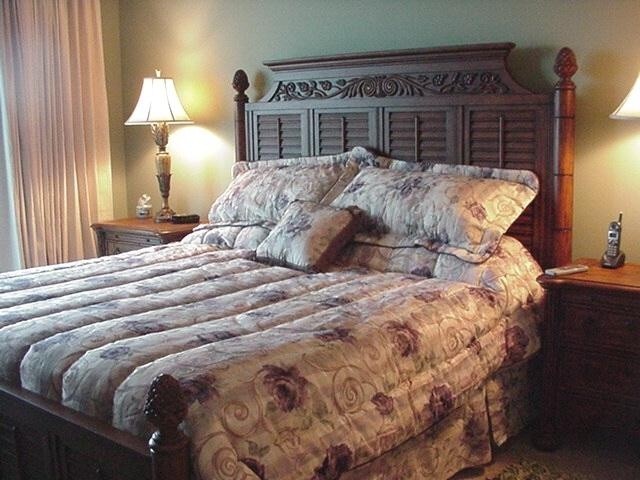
top-left (191, 151), bottom-right (350, 231)
top-left (320, 148), bottom-right (538, 263)
top-left (256, 199), bottom-right (363, 274)
top-left (336, 235), bottom-right (545, 309)
top-left (182, 224), bottom-right (270, 251)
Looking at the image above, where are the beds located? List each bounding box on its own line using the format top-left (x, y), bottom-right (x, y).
top-left (0, 38), bottom-right (579, 480)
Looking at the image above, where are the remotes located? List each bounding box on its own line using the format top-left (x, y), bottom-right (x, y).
top-left (545, 264), bottom-right (588, 277)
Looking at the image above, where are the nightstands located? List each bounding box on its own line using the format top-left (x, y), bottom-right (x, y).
top-left (533, 256), bottom-right (639, 451)
top-left (91, 216), bottom-right (204, 260)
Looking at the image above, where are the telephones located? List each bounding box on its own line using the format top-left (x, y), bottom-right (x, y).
top-left (601, 213), bottom-right (625, 268)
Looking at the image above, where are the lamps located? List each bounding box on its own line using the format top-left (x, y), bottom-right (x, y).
top-left (123, 68), bottom-right (194, 223)
